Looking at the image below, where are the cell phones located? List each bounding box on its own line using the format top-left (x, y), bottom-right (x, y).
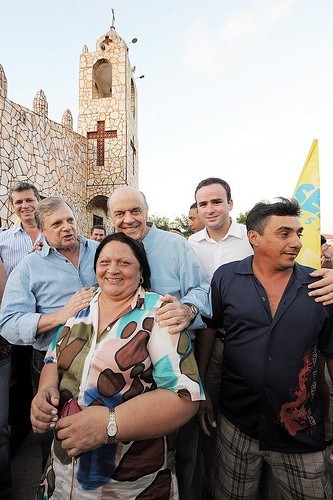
top-left (55, 388), bottom-right (81, 419)
